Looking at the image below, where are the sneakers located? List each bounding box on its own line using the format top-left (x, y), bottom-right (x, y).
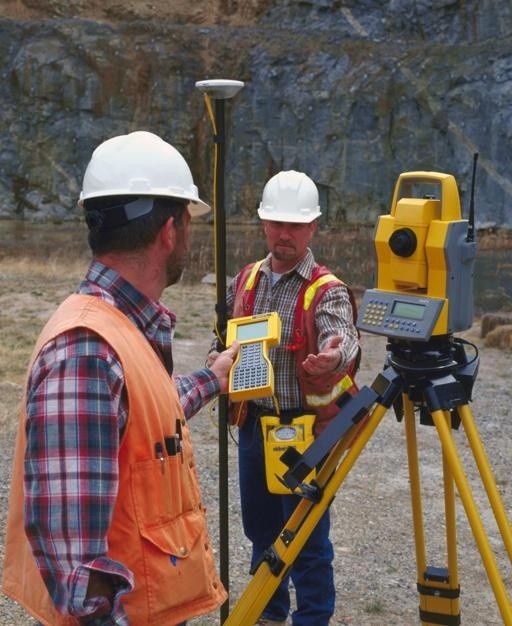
top-left (255, 619), bottom-right (286, 626)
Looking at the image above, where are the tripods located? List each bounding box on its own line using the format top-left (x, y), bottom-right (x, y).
top-left (220, 349), bottom-right (512, 626)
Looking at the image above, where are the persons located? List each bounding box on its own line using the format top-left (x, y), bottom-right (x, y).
top-left (203, 167), bottom-right (360, 626)
top-left (0, 128), bottom-right (240, 626)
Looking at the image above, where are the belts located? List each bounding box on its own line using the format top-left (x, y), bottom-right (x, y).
top-left (252, 406), bottom-right (303, 424)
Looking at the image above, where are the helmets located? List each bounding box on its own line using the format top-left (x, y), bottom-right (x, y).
top-left (257, 170), bottom-right (323, 224)
top-left (77, 131), bottom-right (211, 217)
